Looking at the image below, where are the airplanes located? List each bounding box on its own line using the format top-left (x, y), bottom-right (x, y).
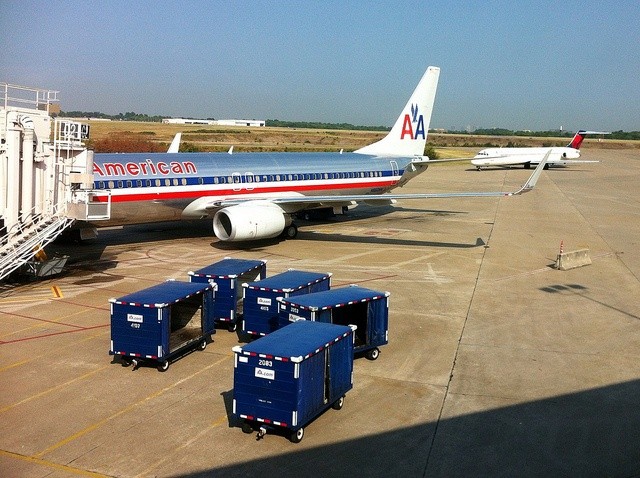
top-left (0, 65), bottom-right (552, 280)
top-left (471, 129), bottom-right (612, 170)
